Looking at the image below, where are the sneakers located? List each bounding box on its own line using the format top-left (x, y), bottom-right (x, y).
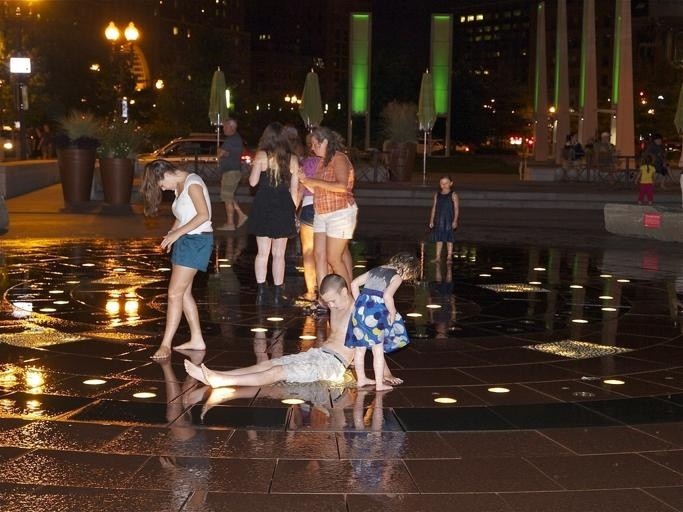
top-left (236, 214), bottom-right (249, 229)
top-left (214, 223), bottom-right (236, 231)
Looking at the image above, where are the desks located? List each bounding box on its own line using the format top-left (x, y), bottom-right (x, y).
top-left (348, 150), bottom-right (391, 185)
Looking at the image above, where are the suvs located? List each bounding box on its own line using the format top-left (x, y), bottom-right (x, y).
top-left (135, 133), bottom-right (253, 162)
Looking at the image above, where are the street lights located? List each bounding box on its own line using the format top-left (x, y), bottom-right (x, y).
top-left (8, 57), bottom-right (32, 161)
top-left (104, 20), bottom-right (140, 124)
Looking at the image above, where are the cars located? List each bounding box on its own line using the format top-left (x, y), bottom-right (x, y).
top-left (418, 137), bottom-right (469, 155)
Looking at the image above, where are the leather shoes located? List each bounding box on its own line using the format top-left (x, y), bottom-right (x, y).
top-left (271, 284), bottom-right (295, 308)
top-left (255, 283), bottom-right (272, 306)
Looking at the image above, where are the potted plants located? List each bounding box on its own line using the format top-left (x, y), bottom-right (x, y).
top-left (96, 117), bottom-right (150, 205)
top-left (50, 116), bottom-right (102, 204)
top-left (379, 100), bottom-right (421, 182)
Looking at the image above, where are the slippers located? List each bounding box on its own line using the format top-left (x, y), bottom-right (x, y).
top-left (297, 295), bottom-right (316, 302)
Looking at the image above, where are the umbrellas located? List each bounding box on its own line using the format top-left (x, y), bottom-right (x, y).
top-left (208, 67), bottom-right (230, 149)
top-left (416, 67), bottom-right (438, 183)
top-left (297, 68), bottom-right (324, 133)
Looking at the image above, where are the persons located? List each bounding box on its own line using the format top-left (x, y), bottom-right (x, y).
top-left (253, 305), bottom-right (285, 363)
top-left (282, 315), bottom-right (331, 480)
top-left (424, 260), bottom-right (457, 348)
top-left (181, 273), bottom-right (405, 388)
top-left (345, 387), bottom-right (415, 488)
top-left (135, 158), bottom-right (215, 360)
top-left (342, 252), bottom-right (422, 392)
top-left (561, 130), bottom-right (584, 161)
top-left (591, 131), bottom-right (623, 165)
top-left (153, 349), bottom-right (216, 511)
top-left (294, 131), bottom-right (355, 302)
top-left (427, 173), bottom-right (459, 265)
top-left (246, 119), bottom-right (299, 310)
top-left (209, 238), bottom-right (249, 342)
top-left (216, 120), bottom-right (249, 231)
top-left (179, 381), bottom-right (365, 411)
top-left (298, 126), bottom-right (360, 317)
top-left (632, 131), bottom-right (683, 211)
